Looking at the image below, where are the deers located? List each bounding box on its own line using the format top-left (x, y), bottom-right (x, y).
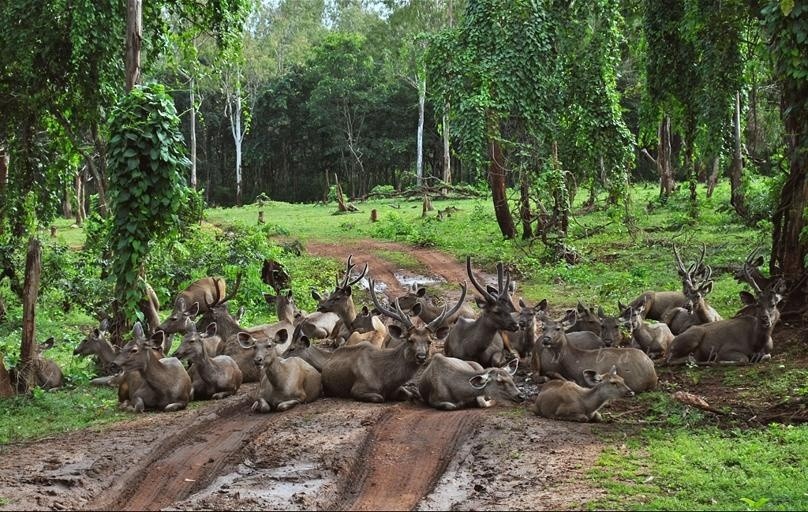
top-left (33, 337), bottom-right (68, 393)
top-left (73, 244), bottom-right (785, 423)
